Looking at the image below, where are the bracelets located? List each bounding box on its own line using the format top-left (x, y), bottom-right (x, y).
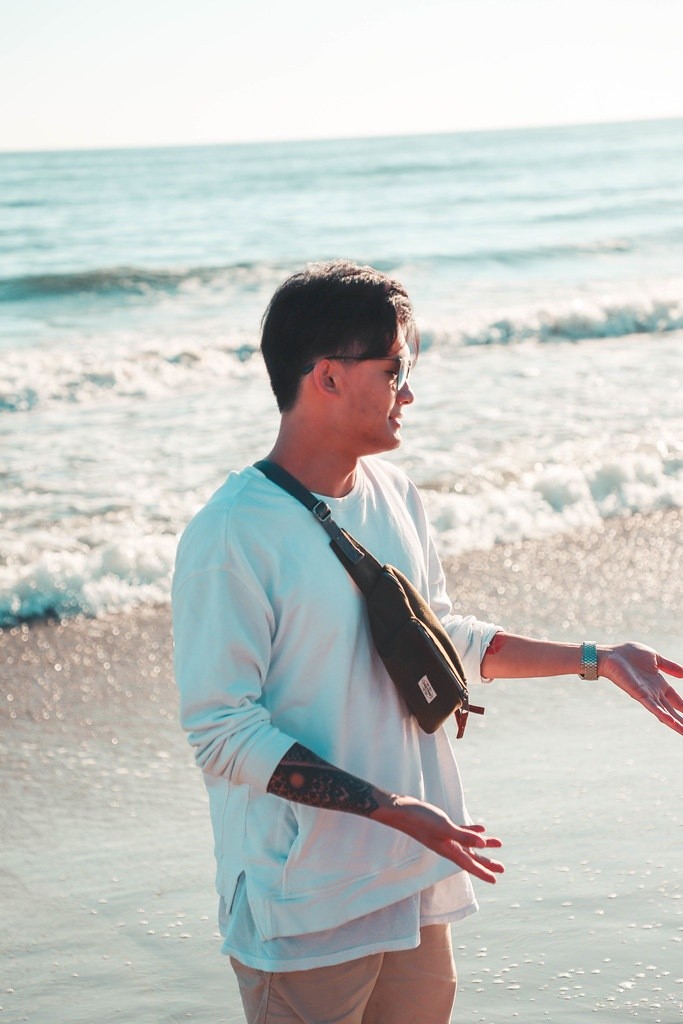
top-left (578, 641), bottom-right (599, 681)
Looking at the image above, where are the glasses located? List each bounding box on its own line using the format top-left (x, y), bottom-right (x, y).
top-left (304, 355), bottom-right (412, 391)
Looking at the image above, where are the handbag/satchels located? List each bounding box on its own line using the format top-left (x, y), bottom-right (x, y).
top-left (252, 457), bottom-right (469, 736)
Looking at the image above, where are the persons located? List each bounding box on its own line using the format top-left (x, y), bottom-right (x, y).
top-left (169, 260), bottom-right (683, 1024)
